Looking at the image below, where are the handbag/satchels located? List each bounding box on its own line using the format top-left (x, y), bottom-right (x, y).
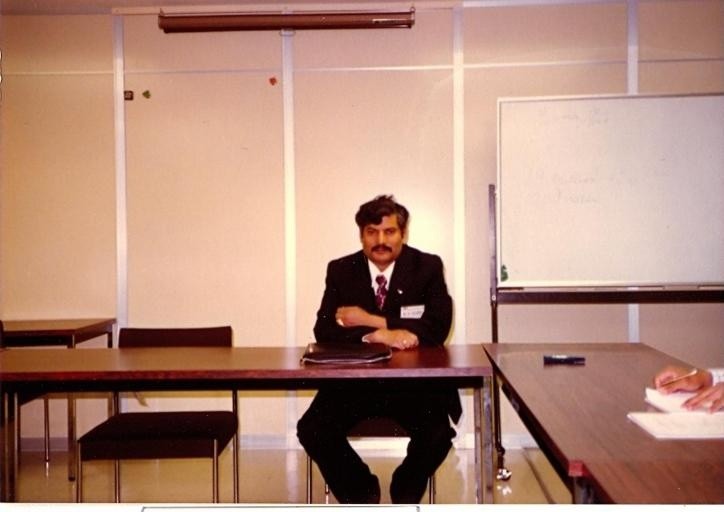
top-left (301, 342), bottom-right (392, 365)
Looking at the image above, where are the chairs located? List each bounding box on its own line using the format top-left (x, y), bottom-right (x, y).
top-left (308, 297), bottom-right (453, 507)
top-left (77, 326), bottom-right (239, 505)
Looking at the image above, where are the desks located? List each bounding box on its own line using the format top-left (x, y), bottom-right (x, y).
top-left (483, 341), bottom-right (707, 503)
top-left (3, 320), bottom-right (117, 481)
top-left (586, 443), bottom-right (723, 502)
top-left (1, 343), bottom-right (497, 504)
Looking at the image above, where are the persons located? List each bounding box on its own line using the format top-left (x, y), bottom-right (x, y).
top-left (655, 364), bottom-right (724, 414)
top-left (297, 195), bottom-right (463, 504)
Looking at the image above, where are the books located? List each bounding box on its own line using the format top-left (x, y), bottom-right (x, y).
top-left (645, 386), bottom-right (713, 411)
top-left (627, 411), bottom-right (724, 439)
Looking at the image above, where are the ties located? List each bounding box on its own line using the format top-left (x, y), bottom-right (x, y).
top-left (376, 275), bottom-right (388, 311)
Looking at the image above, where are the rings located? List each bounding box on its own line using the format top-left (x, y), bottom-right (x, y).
top-left (402, 339), bottom-right (406, 345)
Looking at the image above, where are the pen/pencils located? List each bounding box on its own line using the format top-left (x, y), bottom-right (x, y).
top-left (543, 355), bottom-right (586, 364)
top-left (651, 370), bottom-right (697, 388)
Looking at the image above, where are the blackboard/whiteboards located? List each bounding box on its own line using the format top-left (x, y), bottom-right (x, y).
top-left (496, 92), bottom-right (724, 290)
